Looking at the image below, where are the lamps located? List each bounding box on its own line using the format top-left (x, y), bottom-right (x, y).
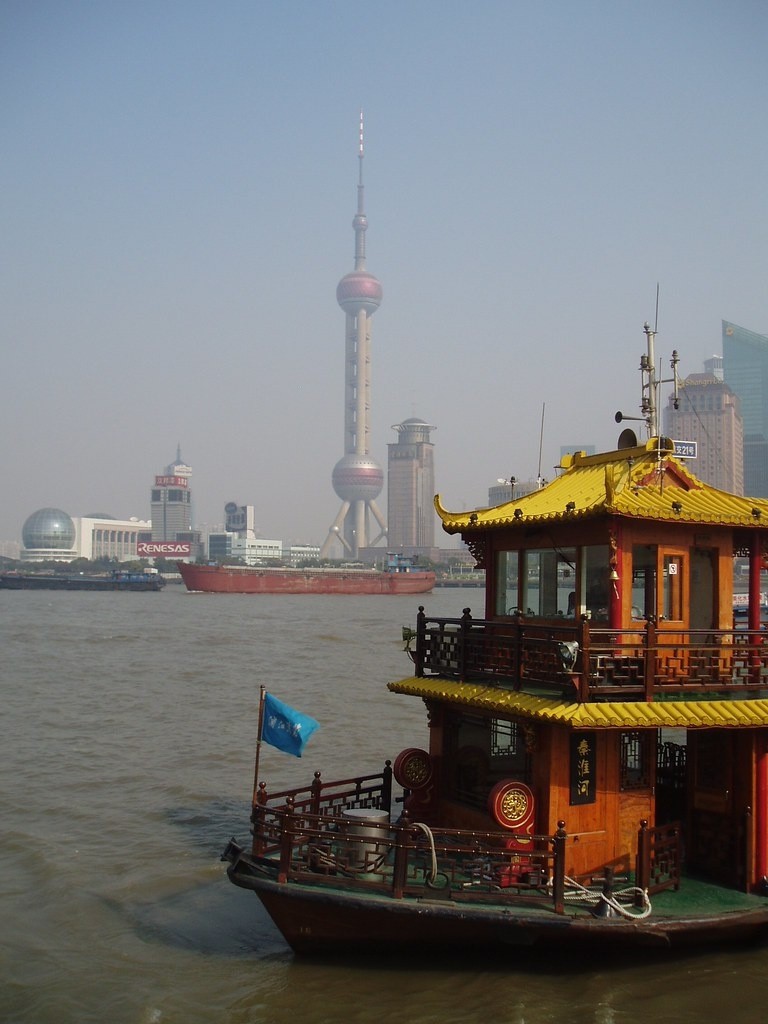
top-left (566, 502), bottom-right (575, 512)
top-left (672, 501), bottom-right (682, 514)
top-left (470, 514), bottom-right (478, 523)
top-left (751, 508), bottom-right (761, 520)
top-left (514, 509), bottom-right (523, 519)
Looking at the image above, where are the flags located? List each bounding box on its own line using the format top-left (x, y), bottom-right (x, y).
top-left (261, 690), bottom-right (320, 759)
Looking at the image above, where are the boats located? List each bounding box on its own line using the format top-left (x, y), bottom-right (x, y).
top-left (0, 569), bottom-right (168, 592)
top-left (177, 553), bottom-right (436, 595)
top-left (221, 280), bottom-right (768, 965)
